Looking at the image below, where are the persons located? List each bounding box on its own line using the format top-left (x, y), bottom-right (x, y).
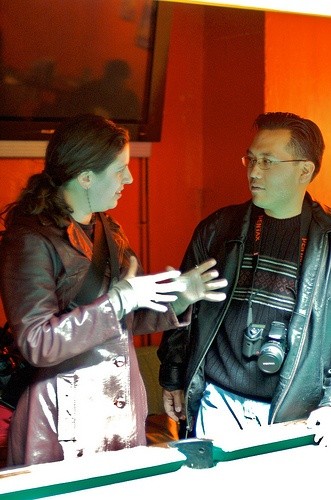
top-left (157, 112), bottom-right (331, 449)
top-left (58, 59), bottom-right (140, 125)
top-left (0, 114), bottom-right (228, 470)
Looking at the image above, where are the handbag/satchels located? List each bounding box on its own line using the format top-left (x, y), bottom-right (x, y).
top-left (0, 328), bottom-right (37, 410)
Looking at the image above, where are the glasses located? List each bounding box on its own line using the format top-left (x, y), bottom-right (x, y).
top-left (241, 153), bottom-right (310, 169)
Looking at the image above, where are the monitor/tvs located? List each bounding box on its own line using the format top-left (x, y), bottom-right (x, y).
top-left (0, 0), bottom-right (171, 142)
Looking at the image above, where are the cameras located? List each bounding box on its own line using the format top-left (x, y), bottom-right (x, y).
top-left (242, 320), bottom-right (288, 374)
